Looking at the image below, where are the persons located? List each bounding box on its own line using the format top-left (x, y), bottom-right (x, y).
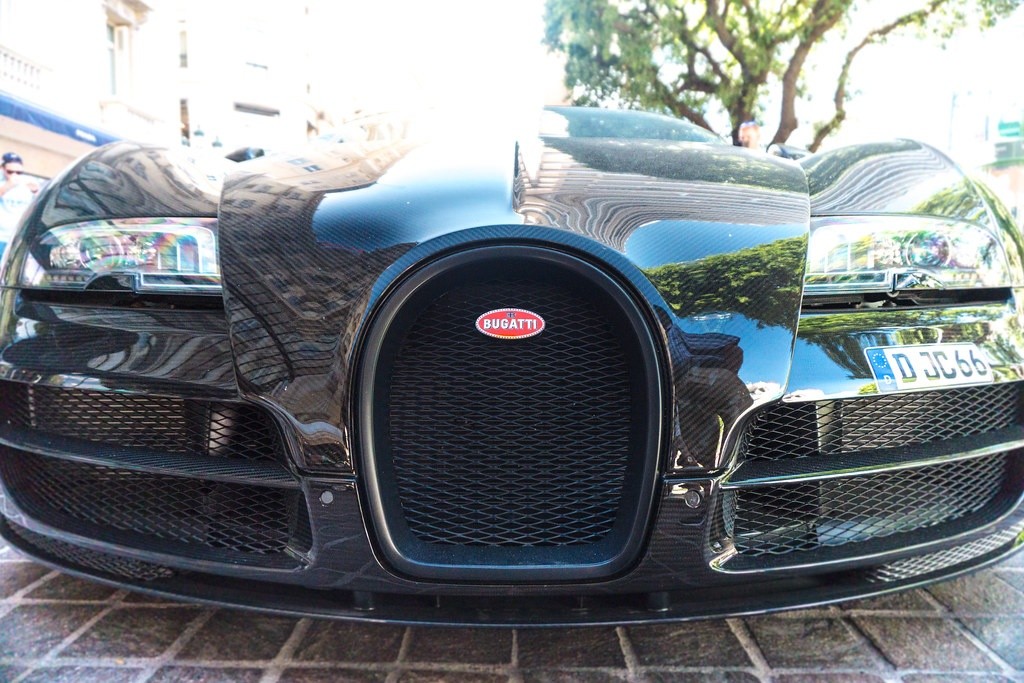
top-left (0, 151), bottom-right (40, 262)
top-left (738, 121), bottom-right (761, 150)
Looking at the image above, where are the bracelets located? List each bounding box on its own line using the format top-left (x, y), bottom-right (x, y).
top-left (30, 189), bottom-right (39, 193)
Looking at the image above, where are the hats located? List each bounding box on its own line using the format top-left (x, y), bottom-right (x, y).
top-left (0, 152), bottom-right (23, 166)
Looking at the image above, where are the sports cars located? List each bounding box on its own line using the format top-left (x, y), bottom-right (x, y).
top-left (0, 107), bottom-right (1024, 630)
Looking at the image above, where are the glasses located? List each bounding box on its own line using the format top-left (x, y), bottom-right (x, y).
top-left (6, 169), bottom-right (22, 175)
